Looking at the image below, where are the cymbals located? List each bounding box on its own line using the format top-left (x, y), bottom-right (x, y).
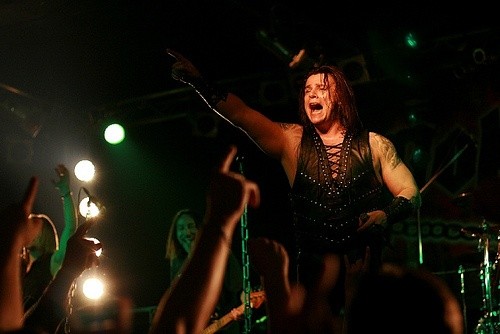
top-left (462, 227), bottom-right (497, 239)
top-left (431, 266), bottom-right (478, 274)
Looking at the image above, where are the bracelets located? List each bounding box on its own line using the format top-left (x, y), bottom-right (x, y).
top-left (60, 191), bottom-right (71, 201)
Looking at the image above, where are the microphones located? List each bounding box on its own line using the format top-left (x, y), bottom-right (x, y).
top-left (83, 186), bottom-right (107, 217)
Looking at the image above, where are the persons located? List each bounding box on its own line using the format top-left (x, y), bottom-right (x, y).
top-left (0, 145), bottom-right (463, 334)
top-left (165, 209), bottom-right (245, 334)
top-left (166, 48), bottom-right (421, 334)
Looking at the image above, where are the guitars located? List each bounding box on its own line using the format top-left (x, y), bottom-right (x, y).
top-left (202, 287), bottom-right (266, 334)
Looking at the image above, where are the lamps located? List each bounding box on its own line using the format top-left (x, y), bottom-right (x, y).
top-left (191, 54), bottom-right (371, 139)
